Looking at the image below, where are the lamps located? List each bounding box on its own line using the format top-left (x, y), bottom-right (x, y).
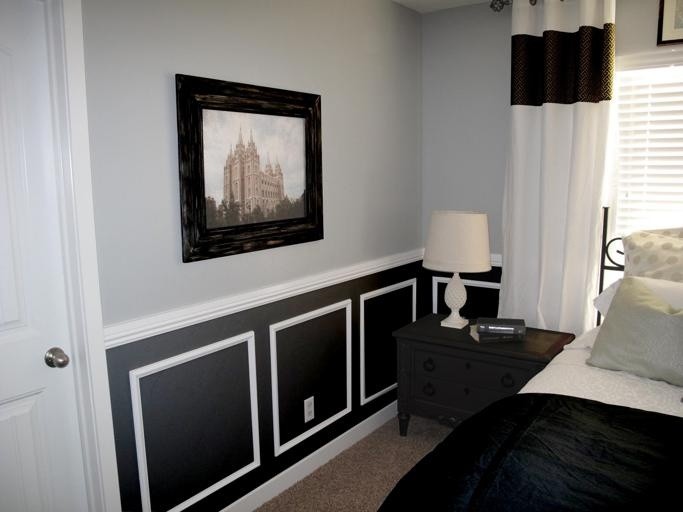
top-left (421, 209), bottom-right (493, 331)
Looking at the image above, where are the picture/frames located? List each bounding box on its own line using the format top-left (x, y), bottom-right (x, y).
top-left (174, 71), bottom-right (325, 263)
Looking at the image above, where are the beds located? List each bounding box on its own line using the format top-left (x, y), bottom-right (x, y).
top-left (372, 207), bottom-right (683, 510)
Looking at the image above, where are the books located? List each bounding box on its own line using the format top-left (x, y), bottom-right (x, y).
top-left (468, 316), bottom-right (528, 345)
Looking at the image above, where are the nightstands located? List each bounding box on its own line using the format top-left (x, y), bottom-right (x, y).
top-left (392, 314), bottom-right (575, 437)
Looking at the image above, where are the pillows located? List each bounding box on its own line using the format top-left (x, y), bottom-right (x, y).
top-left (587, 228), bottom-right (683, 389)
